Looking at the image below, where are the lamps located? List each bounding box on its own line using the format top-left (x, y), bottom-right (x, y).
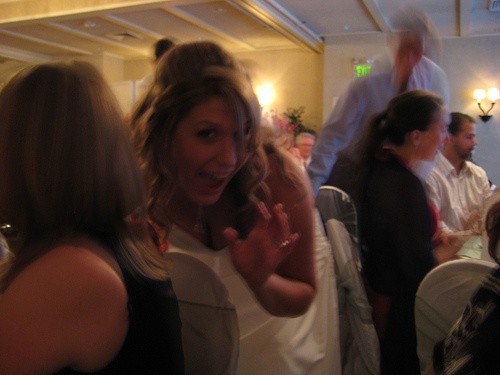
top-left (473, 88), bottom-right (500, 122)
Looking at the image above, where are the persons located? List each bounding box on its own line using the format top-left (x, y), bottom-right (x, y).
top-left (0, 61), bottom-right (186, 375)
top-left (130, 15), bottom-right (500, 375)
top-left (426, 112), bottom-right (492, 260)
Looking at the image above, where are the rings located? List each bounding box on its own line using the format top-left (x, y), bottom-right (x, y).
top-left (281, 241), bottom-right (289, 247)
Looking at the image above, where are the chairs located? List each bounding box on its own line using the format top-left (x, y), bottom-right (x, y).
top-left (315, 185), bottom-right (381, 375)
top-left (413, 258), bottom-right (498, 375)
top-left (161, 250), bottom-right (240, 375)
